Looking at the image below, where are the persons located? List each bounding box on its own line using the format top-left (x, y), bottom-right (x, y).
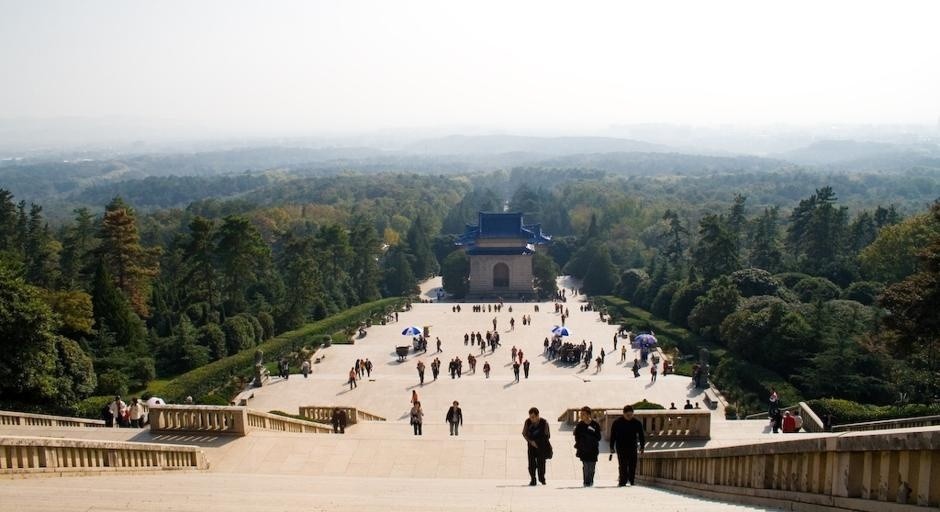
top-left (331, 406), bottom-right (339, 433)
top-left (670, 400), bottom-right (700, 409)
top-left (340, 410), bottom-right (346, 434)
top-left (104, 396), bottom-right (148, 428)
top-left (301, 359), bottom-right (309, 378)
top-left (412, 390), bottom-right (417, 404)
top-left (278, 359), bottom-right (285, 377)
top-left (349, 358), bottom-right (375, 390)
top-left (411, 402), bottom-right (424, 435)
top-left (403, 284), bottom-right (669, 386)
top-left (446, 400), bottom-right (463, 435)
top-left (573, 406), bottom-right (600, 487)
top-left (283, 362), bottom-right (289, 379)
top-left (610, 405), bottom-right (644, 485)
top-left (769, 388), bottom-right (803, 433)
top-left (522, 407), bottom-right (553, 485)
top-left (395, 312), bottom-right (399, 321)
